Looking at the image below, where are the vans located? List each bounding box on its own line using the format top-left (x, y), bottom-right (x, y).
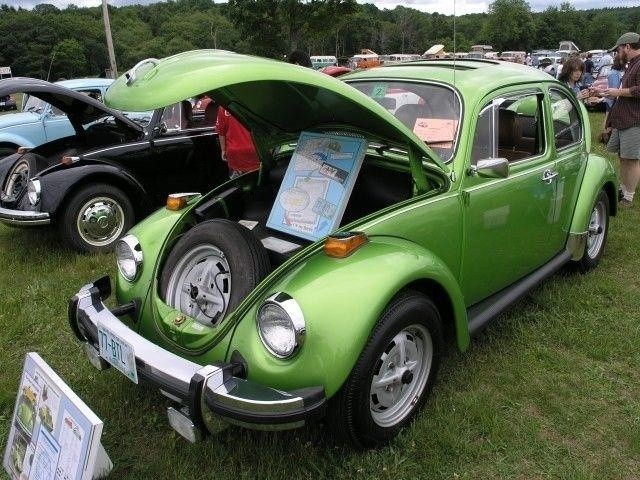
top-left (308, 56), bottom-right (338, 70)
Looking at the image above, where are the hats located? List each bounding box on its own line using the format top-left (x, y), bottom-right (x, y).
top-left (608, 32), bottom-right (640, 53)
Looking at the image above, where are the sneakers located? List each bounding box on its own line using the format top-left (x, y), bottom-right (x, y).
top-left (618, 189), bottom-right (633, 209)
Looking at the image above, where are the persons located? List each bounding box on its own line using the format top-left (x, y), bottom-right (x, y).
top-left (580, 53), bottom-right (596, 84)
top-left (216, 106), bottom-right (259, 177)
top-left (555, 57), bottom-right (570, 80)
top-left (558, 56), bottom-right (599, 101)
top-left (595, 32), bottom-right (640, 209)
top-left (289, 50), bottom-right (314, 69)
top-left (599, 53), bottom-right (627, 142)
top-left (194, 96), bottom-right (216, 111)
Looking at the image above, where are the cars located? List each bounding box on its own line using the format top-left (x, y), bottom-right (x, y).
top-left (64, 46), bottom-right (620, 441)
top-left (0, 80), bottom-right (159, 163)
top-left (0, 75), bottom-right (257, 254)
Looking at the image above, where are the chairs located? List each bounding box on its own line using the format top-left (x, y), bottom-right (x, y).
top-left (394, 104), bottom-right (531, 161)
top-left (174, 100), bottom-right (218, 130)
